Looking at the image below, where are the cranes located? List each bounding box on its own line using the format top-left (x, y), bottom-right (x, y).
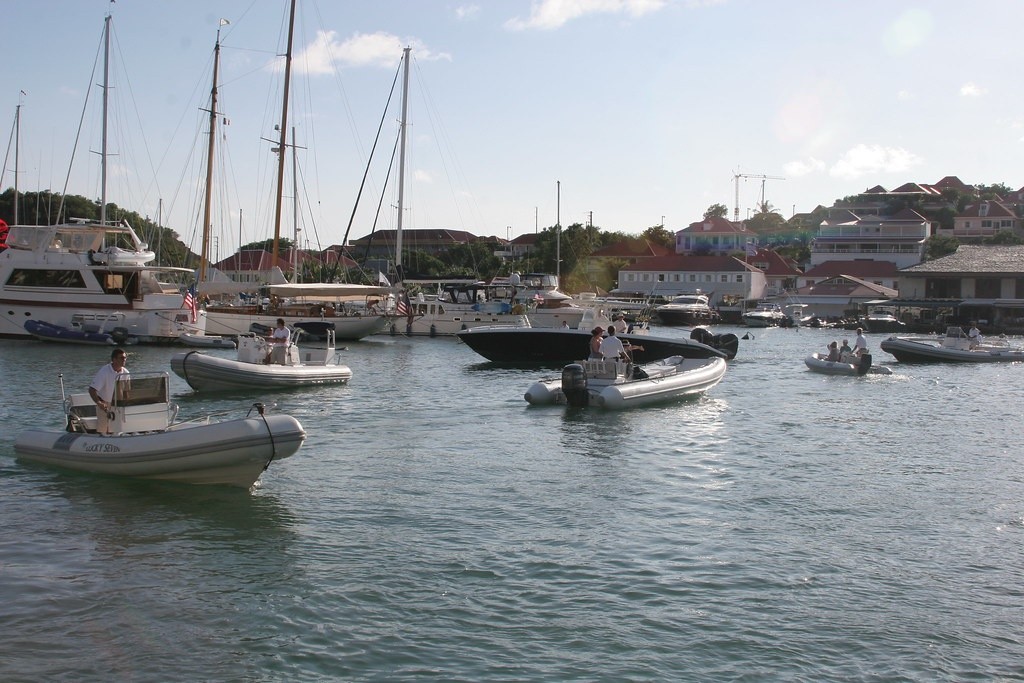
top-left (733, 169), bottom-right (786, 222)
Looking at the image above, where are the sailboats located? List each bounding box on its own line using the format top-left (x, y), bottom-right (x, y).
top-left (148, 1), bottom-right (585, 342)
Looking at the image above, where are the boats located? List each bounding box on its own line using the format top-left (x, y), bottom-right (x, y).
top-left (860, 315), bottom-right (1024, 335)
top-left (804, 352), bottom-right (895, 376)
top-left (0, 218), bottom-right (196, 345)
top-left (170, 317), bottom-right (354, 392)
top-left (740, 302), bottom-right (787, 326)
top-left (523, 355), bottom-right (727, 408)
top-left (653, 290), bottom-right (723, 324)
top-left (877, 324), bottom-right (1024, 363)
top-left (455, 299), bottom-right (739, 365)
top-left (177, 331), bottom-right (238, 349)
top-left (11, 370), bottom-right (309, 489)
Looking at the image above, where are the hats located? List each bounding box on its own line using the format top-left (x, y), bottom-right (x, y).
top-left (616, 314), bottom-right (625, 319)
top-left (621, 340), bottom-right (631, 345)
top-left (595, 327), bottom-right (605, 334)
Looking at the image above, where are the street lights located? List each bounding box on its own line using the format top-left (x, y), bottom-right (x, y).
top-left (662, 216), bottom-right (666, 227)
top-left (506, 226), bottom-right (511, 242)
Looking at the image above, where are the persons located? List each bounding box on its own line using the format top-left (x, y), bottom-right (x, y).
top-left (589, 326), bottom-right (606, 359)
top-left (271, 318), bottom-right (291, 365)
top-left (614, 313), bottom-right (626, 334)
top-left (598, 326), bottom-right (631, 363)
top-left (839, 339), bottom-right (851, 354)
top-left (969, 322), bottom-right (979, 350)
top-left (619, 340), bottom-right (644, 362)
top-left (266, 300), bottom-right (275, 315)
top-left (366, 300), bottom-right (381, 315)
top-left (88, 349), bottom-right (131, 433)
top-left (262, 326), bottom-right (274, 354)
top-left (852, 328), bottom-right (868, 357)
top-left (560, 321), bottom-right (569, 329)
top-left (825, 342), bottom-right (840, 363)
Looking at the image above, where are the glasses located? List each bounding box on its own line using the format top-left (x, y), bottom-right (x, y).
top-left (266, 329), bottom-right (273, 332)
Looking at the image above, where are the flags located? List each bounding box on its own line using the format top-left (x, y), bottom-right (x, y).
top-left (380, 272), bottom-right (390, 286)
top-left (534, 288), bottom-right (544, 304)
top-left (184, 283), bottom-right (197, 322)
top-left (398, 300), bottom-right (410, 315)
